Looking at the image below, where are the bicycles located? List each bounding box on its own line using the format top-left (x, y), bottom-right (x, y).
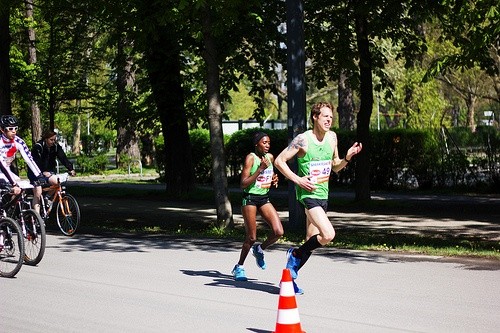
top-left (20, 172), bottom-right (85, 238)
top-left (3, 176), bottom-right (47, 265)
top-left (0, 182), bottom-right (25, 278)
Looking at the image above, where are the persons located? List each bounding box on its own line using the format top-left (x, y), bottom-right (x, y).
top-left (0, 113), bottom-right (49, 250)
top-left (230, 130), bottom-right (284, 282)
top-left (273, 101), bottom-right (363, 294)
top-left (26, 129), bottom-right (76, 227)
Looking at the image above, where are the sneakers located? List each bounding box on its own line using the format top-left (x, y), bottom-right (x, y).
top-left (232, 264), bottom-right (248, 281)
top-left (253, 243), bottom-right (266, 270)
top-left (286, 247), bottom-right (300, 279)
top-left (278, 280), bottom-right (307, 294)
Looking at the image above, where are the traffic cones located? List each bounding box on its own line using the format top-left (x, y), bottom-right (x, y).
top-left (270, 268), bottom-right (306, 333)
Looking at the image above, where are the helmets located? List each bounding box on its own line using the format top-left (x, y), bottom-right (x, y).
top-left (0, 115), bottom-right (18, 128)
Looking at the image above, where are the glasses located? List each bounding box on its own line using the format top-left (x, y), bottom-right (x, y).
top-left (4, 126), bottom-right (20, 131)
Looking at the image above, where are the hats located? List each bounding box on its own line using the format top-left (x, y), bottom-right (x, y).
top-left (253, 130), bottom-right (269, 144)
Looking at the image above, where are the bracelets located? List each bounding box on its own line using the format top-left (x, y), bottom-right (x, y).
top-left (344, 155), bottom-right (350, 162)
